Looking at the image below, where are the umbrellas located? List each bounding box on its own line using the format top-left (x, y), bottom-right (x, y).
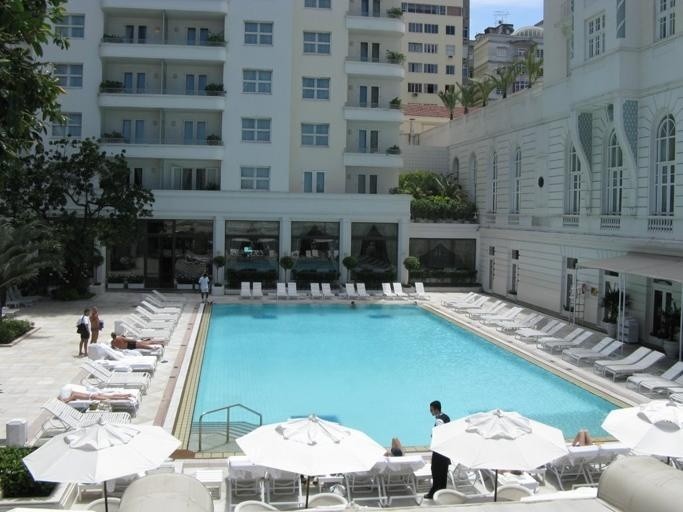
top-left (233, 413), bottom-right (387, 510)
top-left (20, 420), bottom-right (184, 512)
top-left (426, 407), bottom-right (568, 506)
top-left (595, 395), bottom-right (683, 469)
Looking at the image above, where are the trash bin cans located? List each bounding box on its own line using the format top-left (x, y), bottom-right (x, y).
top-left (6, 419), bottom-right (26, 448)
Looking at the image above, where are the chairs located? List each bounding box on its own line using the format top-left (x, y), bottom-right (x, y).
top-left (238, 280), bottom-right (430, 304)
top-left (438, 285), bottom-right (683, 409)
top-left (36, 286), bottom-right (186, 437)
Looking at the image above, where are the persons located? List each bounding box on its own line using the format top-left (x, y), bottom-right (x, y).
top-left (197, 273), bottom-right (210, 304)
top-left (109, 332), bottom-right (153, 349)
top-left (422, 400), bottom-right (451, 499)
top-left (74, 308), bottom-right (90, 357)
top-left (384, 436), bottom-right (405, 457)
top-left (571, 426), bottom-right (595, 447)
top-left (89, 306), bottom-right (103, 344)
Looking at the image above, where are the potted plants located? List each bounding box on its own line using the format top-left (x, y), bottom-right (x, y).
top-left (386, 48), bottom-right (405, 64)
top-left (387, 144), bottom-right (400, 154)
top-left (206, 134), bottom-right (221, 145)
top-left (206, 29), bottom-right (224, 45)
top-left (401, 257), bottom-right (419, 293)
top-left (339, 255), bottom-right (356, 294)
top-left (204, 82), bottom-right (223, 96)
top-left (103, 34), bottom-right (122, 43)
top-left (208, 256), bottom-right (226, 296)
top-left (387, 7), bottom-right (405, 17)
top-left (389, 95), bottom-right (402, 109)
top-left (99, 79), bottom-right (123, 93)
